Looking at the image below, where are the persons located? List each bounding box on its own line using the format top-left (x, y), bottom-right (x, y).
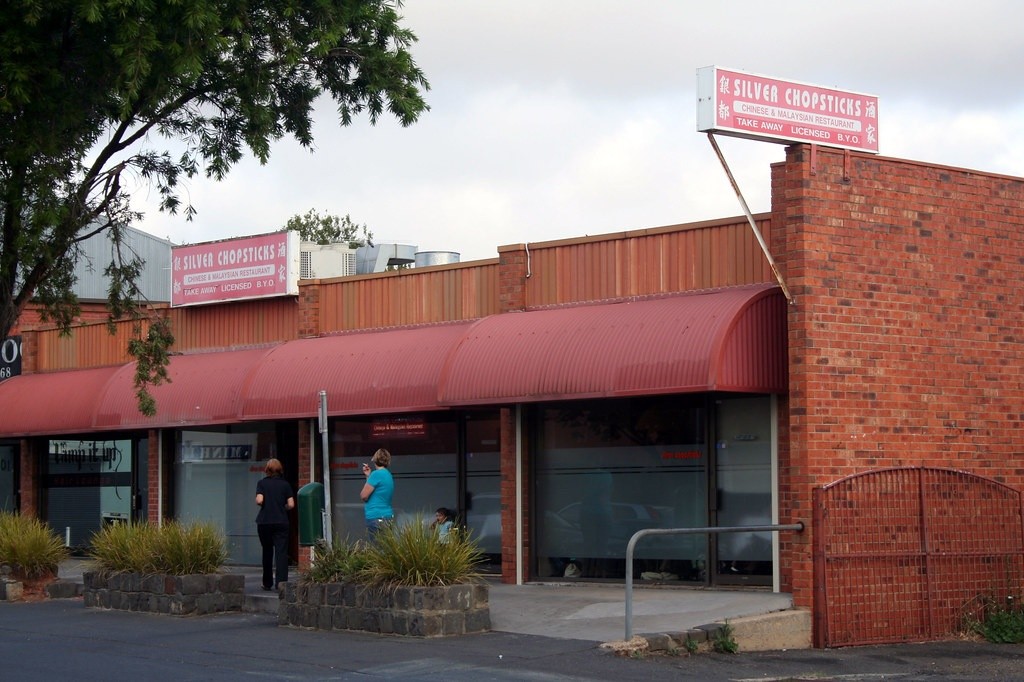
top-left (361, 448), bottom-right (395, 545)
top-left (255, 459), bottom-right (294, 590)
top-left (429, 508), bottom-right (454, 545)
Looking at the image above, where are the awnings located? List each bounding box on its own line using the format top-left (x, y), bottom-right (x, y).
top-left (0, 284), bottom-right (787, 437)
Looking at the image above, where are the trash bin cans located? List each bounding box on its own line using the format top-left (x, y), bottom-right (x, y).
top-left (297, 480), bottom-right (326, 547)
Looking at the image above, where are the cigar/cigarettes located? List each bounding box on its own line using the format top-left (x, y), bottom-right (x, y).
top-left (363, 463), bottom-right (366, 466)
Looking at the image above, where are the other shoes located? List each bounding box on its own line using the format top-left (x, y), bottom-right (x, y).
top-left (261, 585), bottom-right (272, 591)
